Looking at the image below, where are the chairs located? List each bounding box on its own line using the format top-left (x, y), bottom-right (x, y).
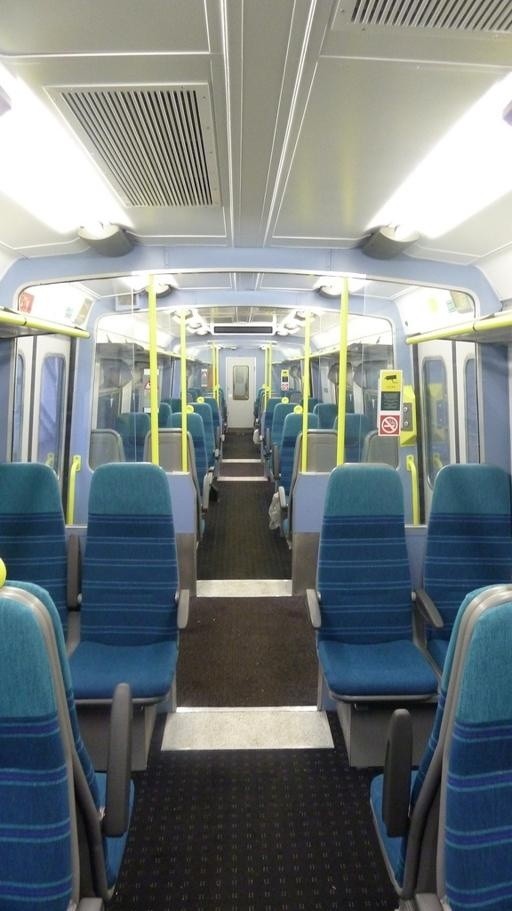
top-left (414, 587), bottom-right (511, 910)
top-left (423, 461), bottom-right (511, 701)
top-left (0, 580), bottom-right (136, 909)
top-left (1, 458), bottom-right (71, 672)
top-left (1, 585), bottom-right (101, 910)
top-left (368, 585), bottom-right (511, 910)
top-left (306, 460), bottom-right (434, 774)
top-left (64, 462), bottom-right (177, 775)
